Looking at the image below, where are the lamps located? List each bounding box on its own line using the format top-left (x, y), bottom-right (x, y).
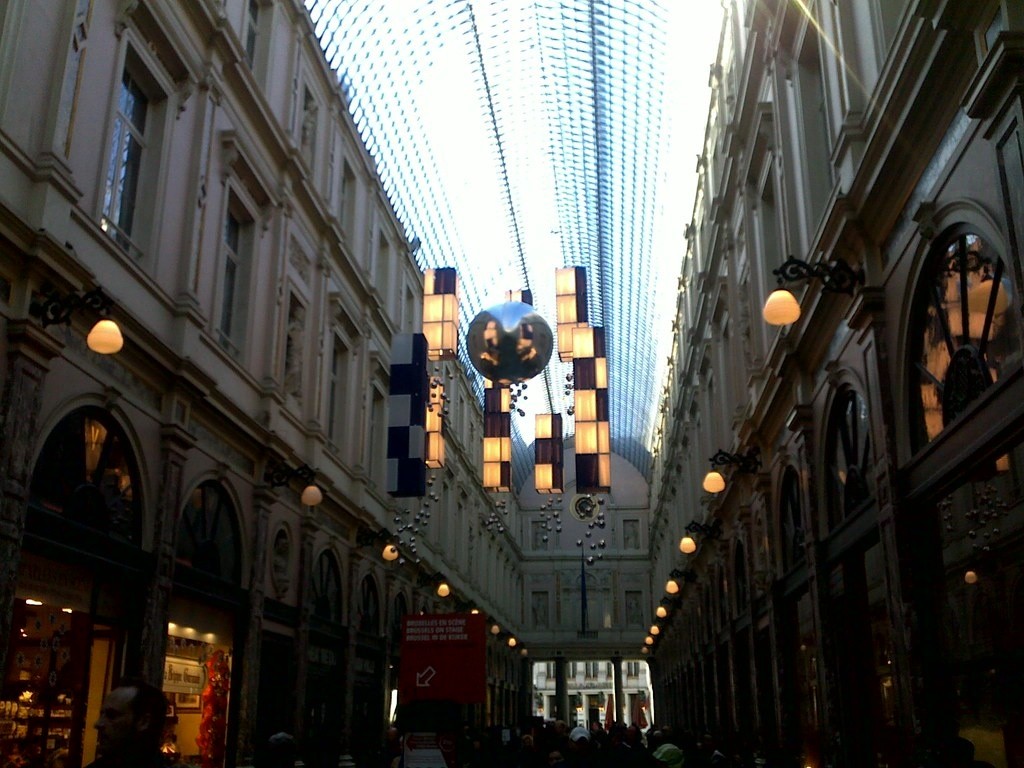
top-left (656, 597), bottom-right (684, 617)
top-left (459, 600), bottom-right (527, 655)
top-left (679, 519), bottom-right (722, 555)
top-left (665, 569), bottom-right (698, 594)
top-left (417, 568), bottom-right (450, 598)
top-left (30, 285), bottom-right (123, 355)
top-left (701, 446), bottom-right (761, 493)
top-left (386, 267), bottom-right (611, 498)
top-left (640, 620), bottom-right (671, 654)
top-left (762, 254), bottom-right (864, 325)
top-left (360, 523), bottom-right (400, 561)
top-left (266, 460), bottom-right (323, 506)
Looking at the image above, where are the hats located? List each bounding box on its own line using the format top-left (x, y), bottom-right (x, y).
top-left (653, 743), bottom-right (685, 767)
top-left (569, 727), bottom-right (591, 741)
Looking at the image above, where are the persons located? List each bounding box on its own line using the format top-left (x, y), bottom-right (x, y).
top-left (462, 720), bottom-right (733, 768)
top-left (83, 680), bottom-right (201, 768)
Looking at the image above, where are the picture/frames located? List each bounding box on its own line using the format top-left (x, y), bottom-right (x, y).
top-left (170, 693), bottom-right (203, 713)
